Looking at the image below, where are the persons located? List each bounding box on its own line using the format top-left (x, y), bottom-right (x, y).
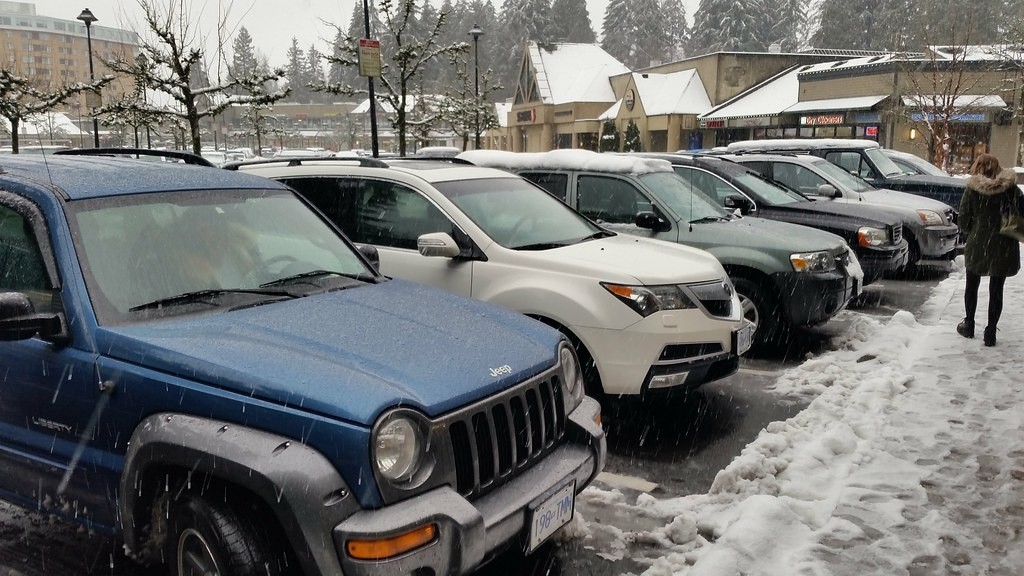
top-left (957, 153), bottom-right (1024, 348)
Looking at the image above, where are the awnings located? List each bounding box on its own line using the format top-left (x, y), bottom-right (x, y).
top-left (782, 93), bottom-right (891, 114)
top-left (903, 95), bottom-right (1007, 112)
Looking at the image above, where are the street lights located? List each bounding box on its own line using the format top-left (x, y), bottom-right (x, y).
top-left (76, 6), bottom-right (101, 146)
top-left (467, 21), bottom-right (486, 150)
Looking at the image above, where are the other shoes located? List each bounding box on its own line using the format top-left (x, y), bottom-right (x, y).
top-left (984, 325), bottom-right (995, 346)
top-left (958, 318), bottom-right (975, 337)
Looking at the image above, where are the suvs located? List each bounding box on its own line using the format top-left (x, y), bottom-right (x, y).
top-left (221, 156), bottom-right (755, 425)
top-left (698, 147), bottom-right (959, 272)
top-left (0, 145), bottom-right (612, 575)
top-left (647, 152), bottom-right (910, 296)
top-left (462, 153), bottom-right (866, 371)
top-left (735, 143), bottom-right (984, 254)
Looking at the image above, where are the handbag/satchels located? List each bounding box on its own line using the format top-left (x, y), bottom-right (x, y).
top-left (1000, 214), bottom-right (1024, 243)
